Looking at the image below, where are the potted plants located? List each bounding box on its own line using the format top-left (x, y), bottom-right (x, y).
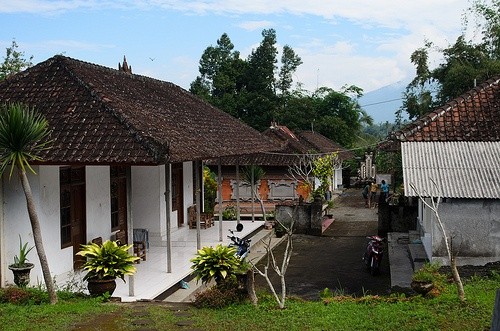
top-left (74, 239), bottom-right (140, 296)
top-left (8, 234), bottom-right (37, 288)
top-left (410, 272), bottom-right (435, 295)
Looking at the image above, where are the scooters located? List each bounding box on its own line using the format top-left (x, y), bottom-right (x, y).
top-left (363, 235), bottom-right (385, 274)
top-left (226, 223), bottom-right (250, 267)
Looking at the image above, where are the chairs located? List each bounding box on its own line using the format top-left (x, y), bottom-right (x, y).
top-left (187, 205), bottom-right (214, 229)
top-left (115, 231), bottom-right (147, 263)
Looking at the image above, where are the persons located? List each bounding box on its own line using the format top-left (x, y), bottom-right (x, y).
top-left (368, 179), bottom-right (379, 208)
top-left (380, 180), bottom-right (389, 193)
top-left (361, 181), bottom-right (372, 208)
